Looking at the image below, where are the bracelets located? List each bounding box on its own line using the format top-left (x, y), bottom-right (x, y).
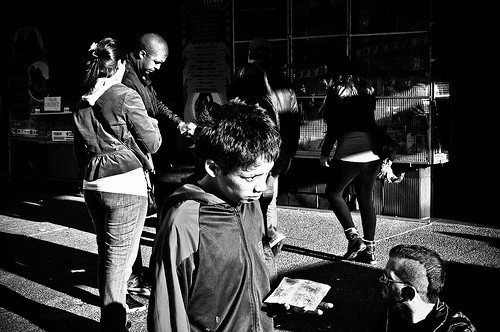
top-left (321, 157), bottom-right (328, 159)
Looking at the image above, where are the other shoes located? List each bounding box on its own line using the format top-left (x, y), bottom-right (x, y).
top-left (268, 232), bottom-right (286, 248)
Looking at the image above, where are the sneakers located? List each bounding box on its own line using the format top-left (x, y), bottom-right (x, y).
top-left (126, 294), bottom-right (147, 314)
top-left (127, 274), bottom-right (152, 296)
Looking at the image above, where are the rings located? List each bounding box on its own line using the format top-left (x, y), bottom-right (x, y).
top-left (322, 163), bottom-right (324, 166)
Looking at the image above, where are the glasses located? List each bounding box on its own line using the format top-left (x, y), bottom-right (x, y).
top-left (382, 273), bottom-right (419, 294)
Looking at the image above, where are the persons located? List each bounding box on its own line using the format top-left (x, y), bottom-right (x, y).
top-left (379, 245), bottom-right (478, 332)
top-left (71, 33), bottom-right (333, 332)
top-left (319, 48), bottom-right (383, 261)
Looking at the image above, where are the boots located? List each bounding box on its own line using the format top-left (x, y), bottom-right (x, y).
top-left (343, 227), bottom-right (366, 262)
top-left (359, 237), bottom-right (376, 261)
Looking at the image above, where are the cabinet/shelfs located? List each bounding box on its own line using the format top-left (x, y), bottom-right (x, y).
top-left (8, 111), bottom-right (86, 184)
top-left (291, 78), bottom-right (450, 165)
